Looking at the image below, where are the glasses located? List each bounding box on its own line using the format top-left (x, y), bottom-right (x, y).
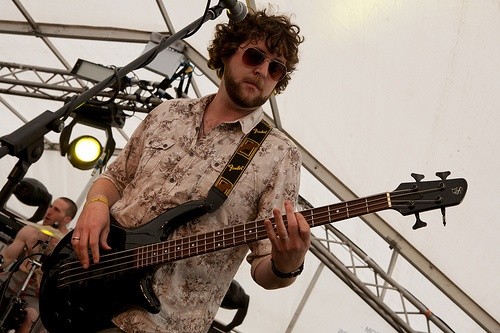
top-left (240, 44), bottom-right (292, 81)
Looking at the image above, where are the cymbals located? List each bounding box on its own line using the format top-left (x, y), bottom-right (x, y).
top-left (13, 216), bottom-right (65, 243)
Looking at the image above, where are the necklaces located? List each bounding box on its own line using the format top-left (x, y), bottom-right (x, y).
top-left (203, 120), bottom-right (208, 139)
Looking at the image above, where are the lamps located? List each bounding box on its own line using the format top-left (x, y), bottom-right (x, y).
top-left (220, 279), bottom-right (250, 332)
top-left (60, 112), bottom-right (116, 170)
top-left (11, 177), bottom-right (52, 222)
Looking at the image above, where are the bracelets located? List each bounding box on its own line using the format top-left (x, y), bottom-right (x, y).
top-left (83, 197), bottom-right (109, 207)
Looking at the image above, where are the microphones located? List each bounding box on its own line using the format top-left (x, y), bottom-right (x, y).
top-left (223, 0), bottom-right (248, 22)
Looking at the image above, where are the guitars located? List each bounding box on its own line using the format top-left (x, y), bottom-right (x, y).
top-left (37, 168), bottom-right (470, 331)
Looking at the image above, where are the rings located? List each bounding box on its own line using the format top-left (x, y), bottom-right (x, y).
top-left (279, 235), bottom-right (289, 240)
top-left (72, 237), bottom-right (80, 240)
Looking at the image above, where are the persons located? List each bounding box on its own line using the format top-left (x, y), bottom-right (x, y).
top-left (0, 198), bottom-right (78, 333)
top-left (71, 5), bottom-right (310, 333)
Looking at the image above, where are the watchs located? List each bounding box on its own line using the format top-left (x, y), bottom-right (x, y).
top-left (270, 254), bottom-right (304, 278)
